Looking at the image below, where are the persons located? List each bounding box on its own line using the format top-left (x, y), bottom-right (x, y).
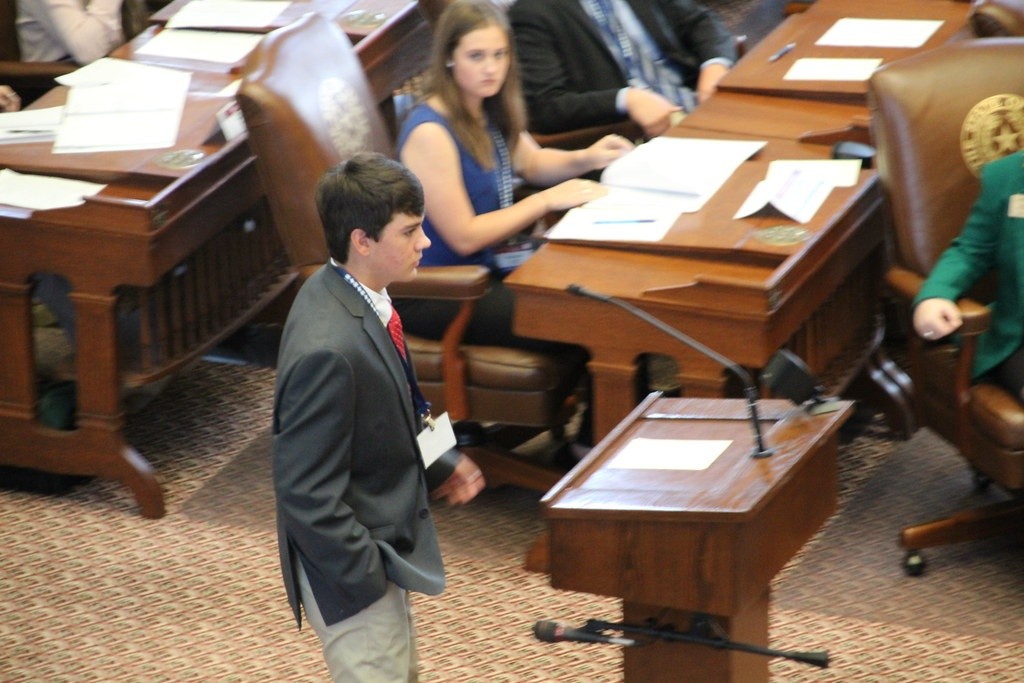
top-left (399, 0), bottom-right (735, 470)
top-left (0, 0), bottom-right (126, 116)
top-left (275, 150), bottom-right (486, 683)
top-left (909, 149), bottom-right (1024, 409)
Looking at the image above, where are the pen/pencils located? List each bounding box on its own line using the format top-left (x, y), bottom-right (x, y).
top-left (768, 42), bottom-right (797, 62)
top-left (9, 129), bottom-right (53, 133)
top-left (595, 219), bottom-right (655, 224)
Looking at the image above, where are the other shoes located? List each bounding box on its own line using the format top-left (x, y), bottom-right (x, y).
top-left (552, 441), bottom-right (581, 474)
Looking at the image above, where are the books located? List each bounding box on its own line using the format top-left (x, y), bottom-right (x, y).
top-left (134, 0), bottom-right (292, 73)
top-left (0, 101), bottom-right (65, 145)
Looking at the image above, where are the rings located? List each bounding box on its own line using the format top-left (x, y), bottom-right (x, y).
top-left (922, 331), bottom-right (933, 339)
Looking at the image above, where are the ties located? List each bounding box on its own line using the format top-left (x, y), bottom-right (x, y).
top-left (588, 0), bottom-right (700, 117)
top-left (388, 305), bottom-right (412, 410)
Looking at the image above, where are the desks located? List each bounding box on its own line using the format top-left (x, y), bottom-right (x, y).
top-left (503, 0), bottom-right (989, 574)
top-left (0, 0), bottom-right (424, 519)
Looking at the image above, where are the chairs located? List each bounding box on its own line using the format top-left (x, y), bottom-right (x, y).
top-left (235, 9), bottom-right (575, 499)
top-left (513, 22), bottom-right (747, 149)
top-left (872, 36), bottom-right (1024, 574)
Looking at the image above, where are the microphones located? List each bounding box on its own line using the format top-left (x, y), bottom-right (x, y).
top-left (566, 284), bottom-right (772, 460)
top-left (535, 621), bottom-right (646, 647)
